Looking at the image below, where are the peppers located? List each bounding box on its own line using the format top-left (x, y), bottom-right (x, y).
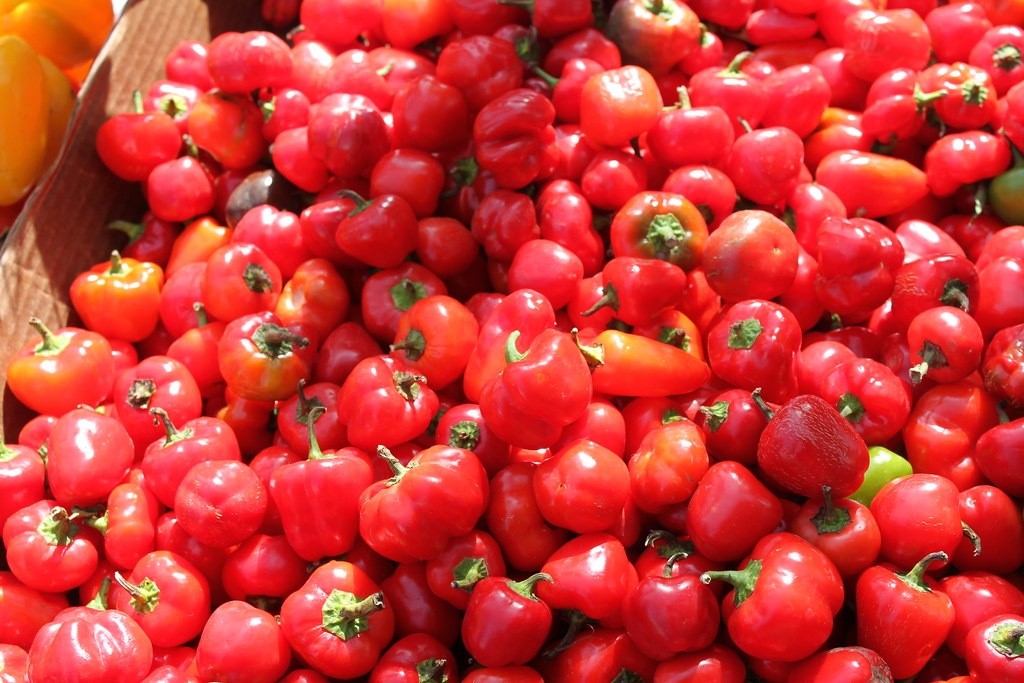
top-left (0, 0), bottom-right (1024, 683)
